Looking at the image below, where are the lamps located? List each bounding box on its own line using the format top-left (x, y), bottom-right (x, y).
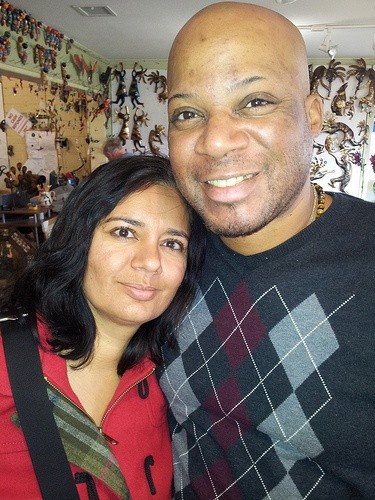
top-left (319, 28), bottom-right (338, 60)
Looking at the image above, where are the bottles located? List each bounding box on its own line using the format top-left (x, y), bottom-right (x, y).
top-left (66, 171), bottom-right (76, 186)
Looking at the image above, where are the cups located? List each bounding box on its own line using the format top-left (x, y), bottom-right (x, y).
top-left (38, 191), bottom-right (55, 206)
top-left (0, 193), bottom-right (12, 210)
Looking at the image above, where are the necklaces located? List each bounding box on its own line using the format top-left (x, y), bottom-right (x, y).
top-left (309, 182), bottom-right (324, 222)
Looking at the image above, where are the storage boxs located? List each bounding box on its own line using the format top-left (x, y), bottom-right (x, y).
top-left (0, 189), bottom-right (17, 208)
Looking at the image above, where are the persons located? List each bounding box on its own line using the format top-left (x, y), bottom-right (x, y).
top-left (146, 2), bottom-right (375, 500)
top-left (0, 156), bottom-right (207, 500)
top-left (102, 138), bottom-right (133, 161)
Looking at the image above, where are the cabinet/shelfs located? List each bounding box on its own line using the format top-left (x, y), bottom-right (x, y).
top-left (0, 205), bottom-right (54, 249)
